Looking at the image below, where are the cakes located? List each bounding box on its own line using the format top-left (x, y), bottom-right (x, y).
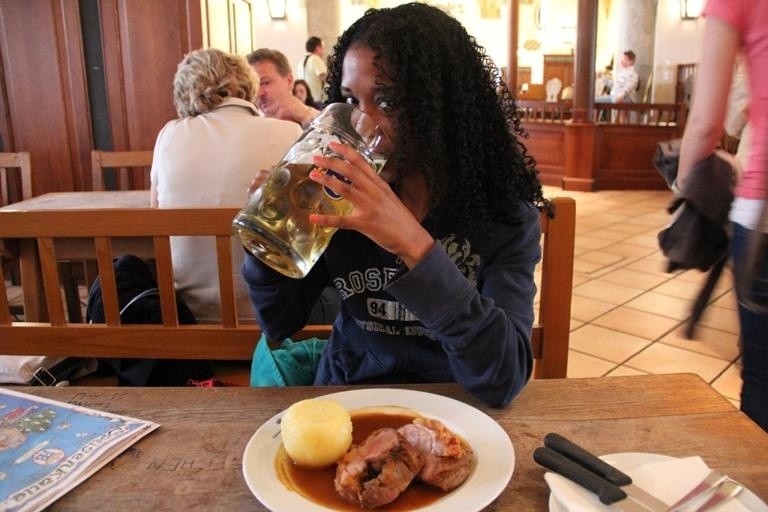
top-left (280, 399), bottom-right (352, 469)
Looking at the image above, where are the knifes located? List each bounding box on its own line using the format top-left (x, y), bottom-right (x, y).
top-left (530, 444), bottom-right (654, 512)
top-left (543, 429), bottom-right (678, 512)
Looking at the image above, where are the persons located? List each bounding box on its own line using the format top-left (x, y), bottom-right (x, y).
top-left (609, 51), bottom-right (639, 122)
top-left (601, 66), bottom-right (611, 123)
top-left (240, 2), bottom-right (556, 410)
top-left (293, 80), bottom-right (324, 111)
top-left (244, 48), bottom-right (319, 129)
top-left (671, 1), bottom-right (767, 433)
top-left (150, 48), bottom-right (342, 324)
top-left (305, 36), bottom-right (330, 110)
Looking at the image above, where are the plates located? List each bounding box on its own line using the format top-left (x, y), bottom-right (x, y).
top-left (241, 385), bottom-right (516, 511)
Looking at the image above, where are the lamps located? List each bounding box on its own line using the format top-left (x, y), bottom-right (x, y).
top-left (266, 0), bottom-right (288, 21)
top-left (679, 0), bottom-right (699, 20)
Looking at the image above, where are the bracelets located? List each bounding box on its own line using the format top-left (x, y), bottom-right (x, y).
top-left (672, 180), bottom-right (690, 198)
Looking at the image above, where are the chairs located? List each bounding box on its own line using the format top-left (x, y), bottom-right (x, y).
top-left (0, 151), bottom-right (33, 208)
top-left (82, 146), bottom-right (156, 294)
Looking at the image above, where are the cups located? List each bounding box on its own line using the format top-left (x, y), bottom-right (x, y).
top-left (230, 101), bottom-right (391, 279)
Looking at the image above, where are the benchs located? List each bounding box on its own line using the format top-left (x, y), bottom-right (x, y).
top-left (0, 195), bottom-right (580, 377)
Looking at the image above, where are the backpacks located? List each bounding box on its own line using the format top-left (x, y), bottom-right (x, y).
top-left (80, 253), bottom-right (218, 386)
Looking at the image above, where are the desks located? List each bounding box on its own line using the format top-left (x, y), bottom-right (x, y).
top-left (2, 359), bottom-right (765, 512)
top-left (0, 185), bottom-right (165, 326)
